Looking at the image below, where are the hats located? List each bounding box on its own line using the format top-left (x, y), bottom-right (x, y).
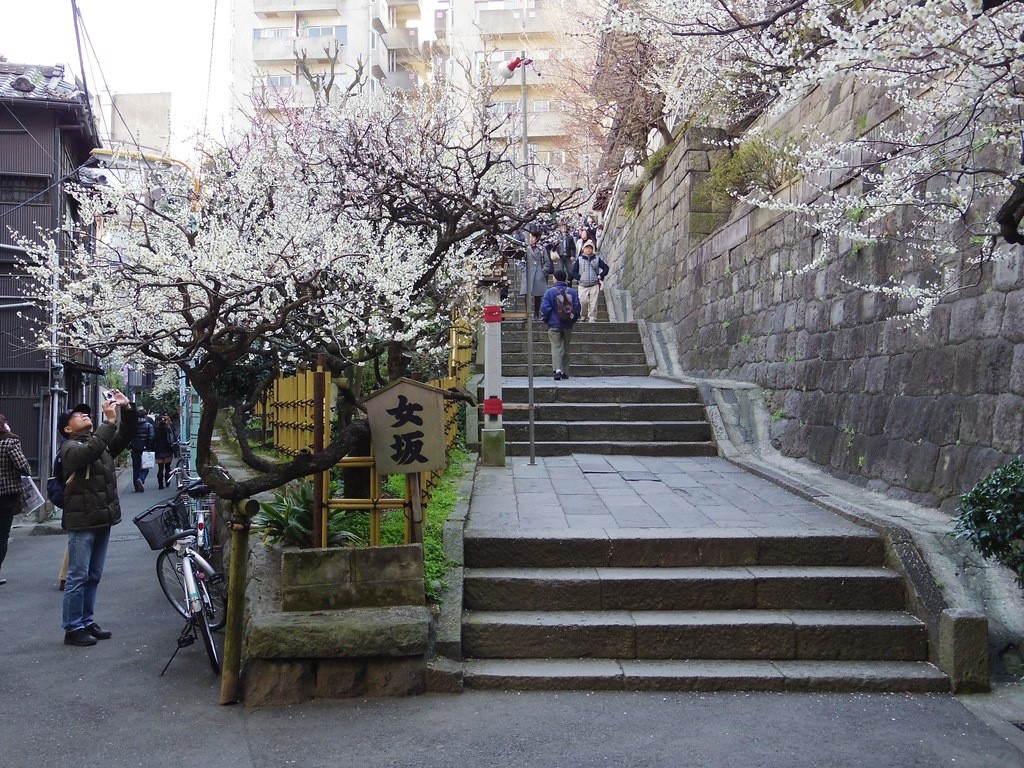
top-left (584, 242), bottom-right (594, 248)
top-left (58, 404), bottom-right (91, 433)
top-left (554, 270), bottom-right (566, 281)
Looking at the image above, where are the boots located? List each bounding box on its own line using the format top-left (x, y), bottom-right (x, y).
top-left (165, 472), bottom-right (170, 487)
top-left (157, 472), bottom-right (165, 490)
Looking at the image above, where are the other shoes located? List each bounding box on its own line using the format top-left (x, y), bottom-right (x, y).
top-left (562, 372), bottom-right (569, 379)
top-left (64, 628), bottom-right (97, 646)
top-left (589, 319), bottom-right (595, 323)
top-left (534, 315), bottom-right (539, 319)
top-left (83, 622), bottom-right (111, 640)
top-left (136, 479), bottom-right (144, 493)
top-left (580, 316), bottom-right (586, 321)
top-left (0, 580), bottom-right (6, 585)
top-left (554, 372), bottom-right (561, 380)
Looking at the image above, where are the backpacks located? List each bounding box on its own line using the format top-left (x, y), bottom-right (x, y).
top-left (47, 441), bottom-right (85, 509)
top-left (553, 286), bottom-right (573, 320)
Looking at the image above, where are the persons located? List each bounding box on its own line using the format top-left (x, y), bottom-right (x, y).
top-left (137, 406), bottom-right (157, 451)
top-left (0, 414), bottom-right (32, 587)
top-left (571, 213), bottom-right (603, 256)
top-left (553, 224), bottom-right (576, 280)
top-left (576, 230), bottom-right (595, 257)
top-left (126, 410), bottom-right (154, 493)
top-left (540, 270), bottom-right (581, 380)
top-left (155, 416), bottom-right (173, 489)
top-left (519, 231), bottom-right (551, 320)
top-left (169, 417), bottom-right (178, 442)
top-left (58, 389), bottom-right (138, 646)
top-left (58, 544), bottom-right (69, 590)
top-left (571, 239), bottom-right (609, 322)
top-left (165, 416), bottom-right (175, 443)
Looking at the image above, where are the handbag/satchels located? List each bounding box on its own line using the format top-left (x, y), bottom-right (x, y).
top-left (19, 475), bottom-right (46, 517)
top-left (141, 449), bottom-right (155, 468)
top-left (170, 442), bottom-right (178, 453)
top-left (550, 248), bottom-right (560, 261)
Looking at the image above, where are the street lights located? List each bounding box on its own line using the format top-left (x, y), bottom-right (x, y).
top-left (496, 48), bottom-right (538, 466)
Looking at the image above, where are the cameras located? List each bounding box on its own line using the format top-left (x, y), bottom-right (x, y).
top-left (102, 391), bottom-right (116, 404)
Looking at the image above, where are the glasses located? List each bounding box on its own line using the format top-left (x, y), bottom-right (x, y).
top-left (71, 413), bottom-right (88, 418)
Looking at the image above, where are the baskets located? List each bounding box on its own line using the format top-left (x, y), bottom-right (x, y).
top-left (132, 497), bottom-right (189, 550)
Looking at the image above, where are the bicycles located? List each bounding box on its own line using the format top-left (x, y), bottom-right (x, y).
top-left (163, 437), bottom-right (231, 576)
top-left (131, 478), bottom-right (229, 678)
